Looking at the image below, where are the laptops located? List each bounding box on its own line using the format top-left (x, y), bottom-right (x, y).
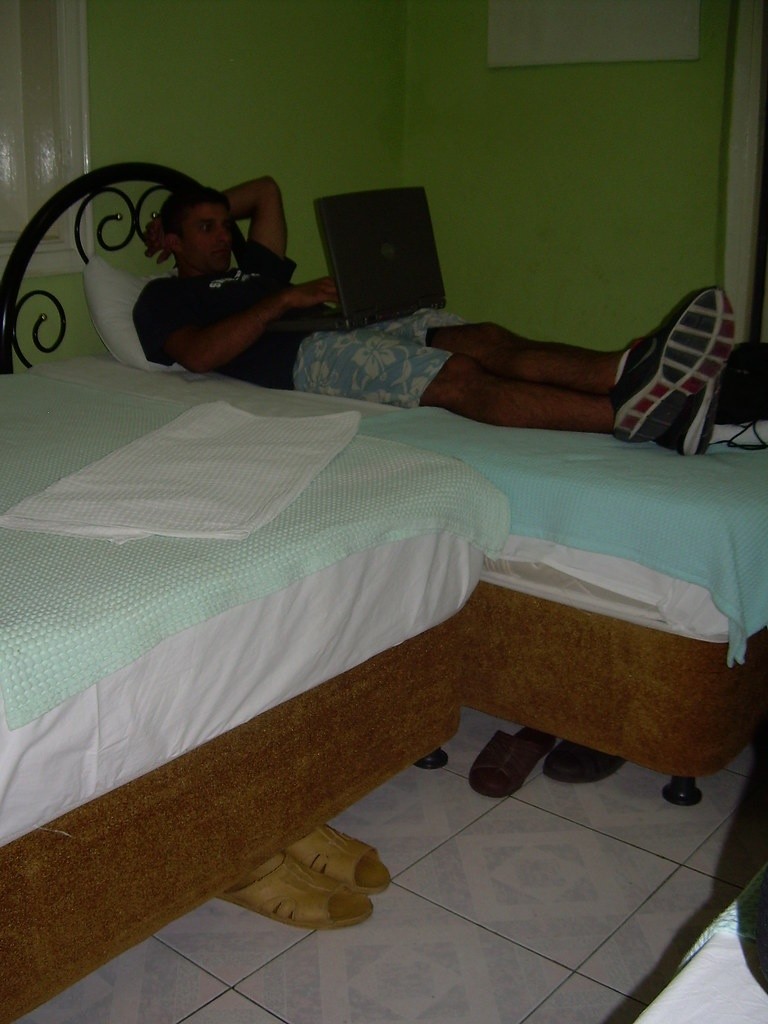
top-left (267, 187), bottom-right (446, 336)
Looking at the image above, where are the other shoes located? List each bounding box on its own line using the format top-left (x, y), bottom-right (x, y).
top-left (609, 285), bottom-right (734, 443)
top-left (414, 748), bottom-right (448, 769)
top-left (652, 372), bottom-right (721, 456)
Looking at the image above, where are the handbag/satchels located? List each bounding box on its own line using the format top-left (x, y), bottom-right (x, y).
top-left (715, 342), bottom-right (768, 425)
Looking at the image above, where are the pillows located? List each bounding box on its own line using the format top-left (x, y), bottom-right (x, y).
top-left (82, 256), bottom-right (185, 375)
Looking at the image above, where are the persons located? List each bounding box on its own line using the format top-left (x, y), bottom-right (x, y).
top-left (131, 176), bottom-right (735, 459)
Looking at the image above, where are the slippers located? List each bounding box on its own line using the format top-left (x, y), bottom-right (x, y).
top-left (469, 726), bottom-right (555, 798)
top-left (544, 740), bottom-right (627, 782)
top-left (216, 852), bottom-right (373, 929)
top-left (285, 824), bottom-right (390, 893)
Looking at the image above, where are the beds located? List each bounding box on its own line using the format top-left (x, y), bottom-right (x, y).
top-left (0, 365), bottom-right (510, 1024)
top-left (0, 161), bottom-right (768, 808)
